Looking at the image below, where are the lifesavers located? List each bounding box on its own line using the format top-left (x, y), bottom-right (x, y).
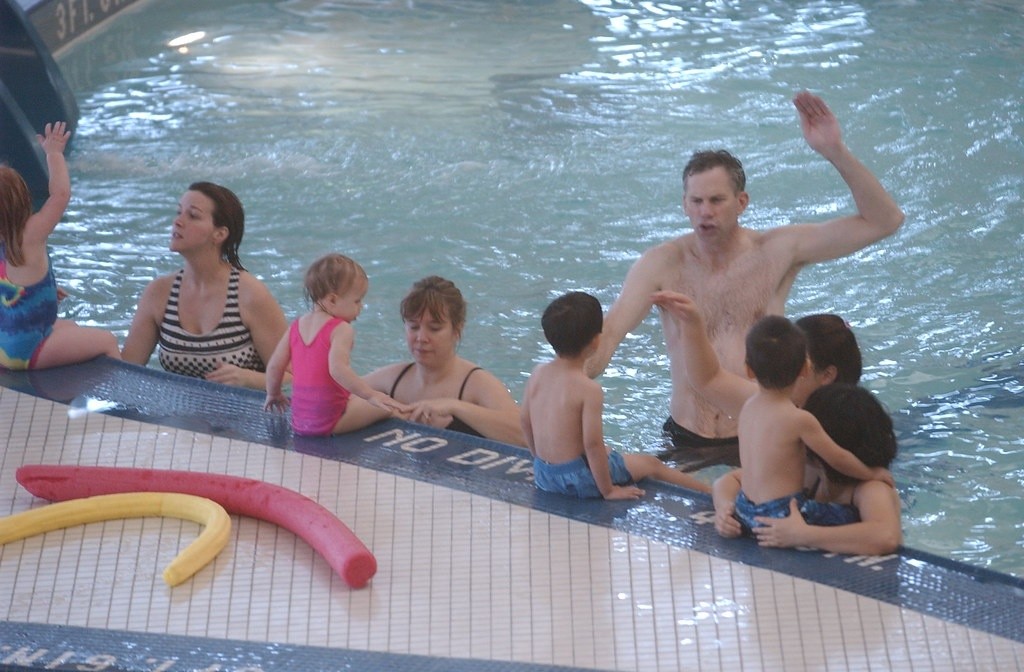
top-left (0, 463), bottom-right (376, 589)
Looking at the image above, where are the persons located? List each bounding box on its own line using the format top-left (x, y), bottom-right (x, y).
top-left (653, 292), bottom-right (901, 555)
top-left (122, 182), bottom-right (293, 390)
top-left (265, 253), bottom-right (453, 437)
top-left (521, 291), bottom-right (713, 502)
top-left (584, 89), bottom-right (905, 449)
top-left (359, 276), bottom-right (528, 447)
top-left (0, 121), bottom-right (121, 371)
